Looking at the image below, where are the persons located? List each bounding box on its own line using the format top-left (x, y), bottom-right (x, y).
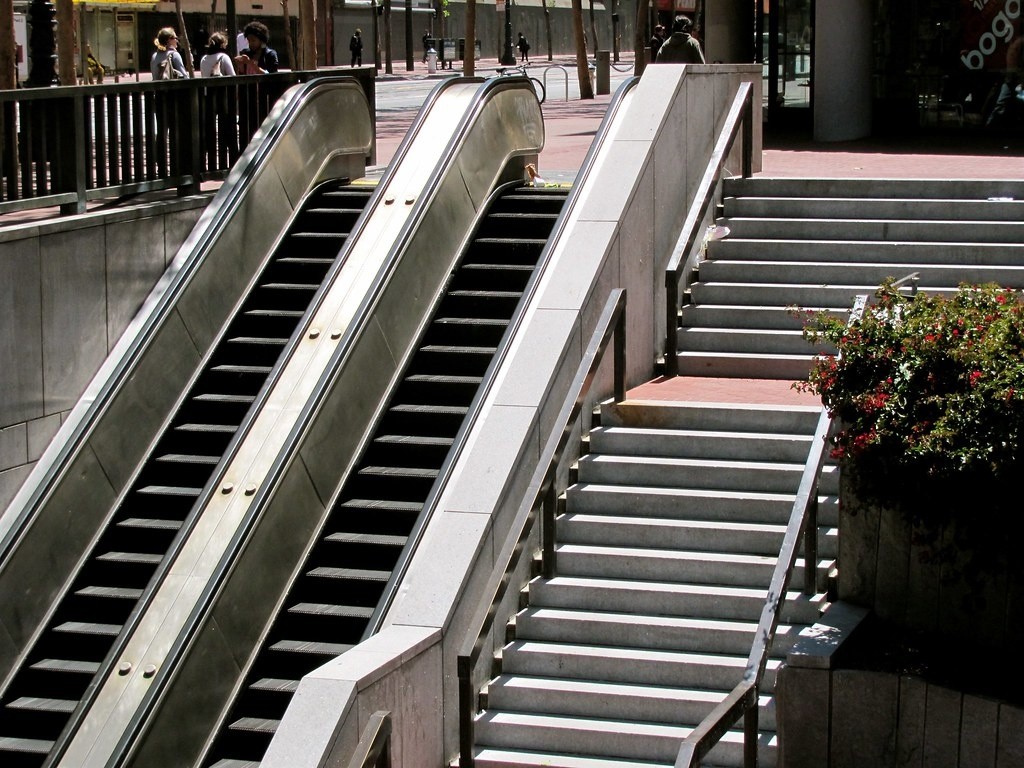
top-left (350, 29), bottom-right (363, 67)
top-left (46, 44), bottom-right (59, 77)
top-left (421, 29), bottom-right (432, 63)
top-left (86, 44), bottom-right (104, 85)
top-left (516, 32), bottom-right (530, 62)
top-left (150, 22), bottom-right (278, 129)
top-left (651, 15), bottom-right (705, 64)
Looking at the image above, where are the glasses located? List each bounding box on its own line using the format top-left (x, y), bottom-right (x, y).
top-left (172, 36), bottom-right (178, 40)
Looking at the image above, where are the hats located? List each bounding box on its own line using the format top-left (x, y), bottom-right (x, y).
top-left (655, 25), bottom-right (665, 32)
top-left (356, 29), bottom-right (361, 32)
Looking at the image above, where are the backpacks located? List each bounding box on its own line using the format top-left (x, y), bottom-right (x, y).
top-left (524, 37), bottom-right (529, 47)
top-left (350, 36), bottom-right (358, 50)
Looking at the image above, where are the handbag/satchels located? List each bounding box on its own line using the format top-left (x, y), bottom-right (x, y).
top-left (210, 55), bottom-right (224, 77)
top-left (157, 51), bottom-right (183, 80)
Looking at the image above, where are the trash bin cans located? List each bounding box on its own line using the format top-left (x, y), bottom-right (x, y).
top-left (426, 38), bottom-right (456, 61)
top-left (459, 38), bottom-right (482, 60)
